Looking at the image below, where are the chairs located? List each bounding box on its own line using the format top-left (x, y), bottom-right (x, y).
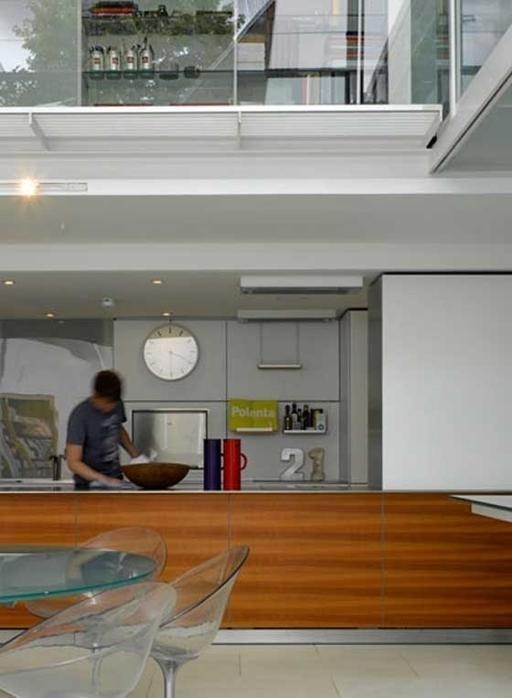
top-left (74, 543), bottom-right (251, 697)
top-left (24, 526), bottom-right (169, 698)
top-left (2, 581), bottom-right (175, 698)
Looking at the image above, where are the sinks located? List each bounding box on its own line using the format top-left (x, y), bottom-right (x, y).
top-left (15, 478), bottom-right (73, 484)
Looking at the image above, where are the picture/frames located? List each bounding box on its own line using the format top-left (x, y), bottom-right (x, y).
top-left (0, 393), bottom-right (59, 482)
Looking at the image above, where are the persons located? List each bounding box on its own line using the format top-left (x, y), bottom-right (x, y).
top-left (65, 370), bottom-right (144, 488)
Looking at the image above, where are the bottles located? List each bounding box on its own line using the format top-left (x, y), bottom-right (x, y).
top-left (279, 402), bottom-right (327, 432)
top-left (83, 37), bottom-right (203, 82)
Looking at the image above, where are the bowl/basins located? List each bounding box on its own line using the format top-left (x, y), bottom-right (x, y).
top-left (115, 461), bottom-right (192, 490)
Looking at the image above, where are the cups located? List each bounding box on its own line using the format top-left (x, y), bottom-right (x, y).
top-left (203, 438), bottom-right (225, 491)
top-left (224, 437), bottom-right (247, 490)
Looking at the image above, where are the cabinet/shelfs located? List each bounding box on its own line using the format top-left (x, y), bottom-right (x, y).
top-left (235, 363), bottom-right (326, 434)
top-left (80, 0), bottom-right (450, 106)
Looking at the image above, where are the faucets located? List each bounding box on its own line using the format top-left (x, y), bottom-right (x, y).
top-left (48, 454), bottom-right (66, 480)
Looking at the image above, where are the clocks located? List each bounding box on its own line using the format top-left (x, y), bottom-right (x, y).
top-left (142, 322), bottom-right (201, 382)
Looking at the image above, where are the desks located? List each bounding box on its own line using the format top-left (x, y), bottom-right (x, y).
top-left (1, 543), bottom-right (158, 603)
